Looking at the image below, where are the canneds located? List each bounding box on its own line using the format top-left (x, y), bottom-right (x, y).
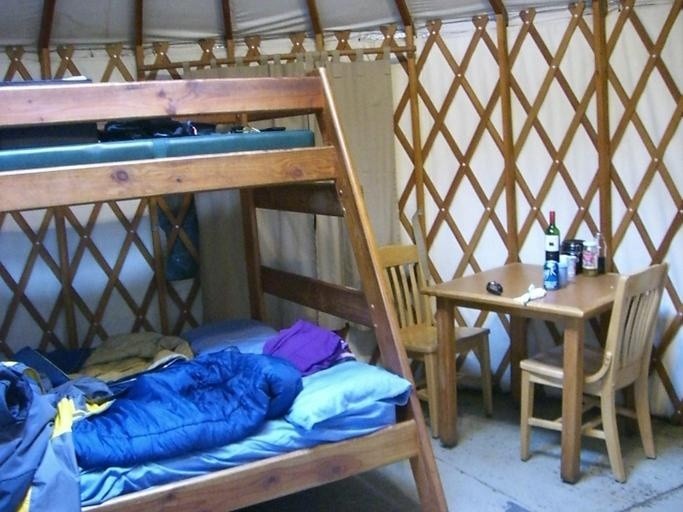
top-left (543, 260), bottom-right (560, 291)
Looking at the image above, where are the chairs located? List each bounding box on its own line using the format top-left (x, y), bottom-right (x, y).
top-left (371, 237), bottom-right (495, 440)
top-left (511, 262), bottom-right (672, 485)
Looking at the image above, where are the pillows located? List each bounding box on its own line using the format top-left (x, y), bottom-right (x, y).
top-left (191, 311), bottom-right (403, 435)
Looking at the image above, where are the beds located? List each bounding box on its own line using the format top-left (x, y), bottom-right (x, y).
top-left (0, 65), bottom-right (449, 511)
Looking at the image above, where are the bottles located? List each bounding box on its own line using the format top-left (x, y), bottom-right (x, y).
top-left (545, 211), bottom-right (559, 262)
top-left (594, 232), bottom-right (606, 275)
top-left (543, 254), bottom-right (577, 290)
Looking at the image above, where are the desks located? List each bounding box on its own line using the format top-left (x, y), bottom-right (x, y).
top-left (411, 262), bottom-right (635, 483)
